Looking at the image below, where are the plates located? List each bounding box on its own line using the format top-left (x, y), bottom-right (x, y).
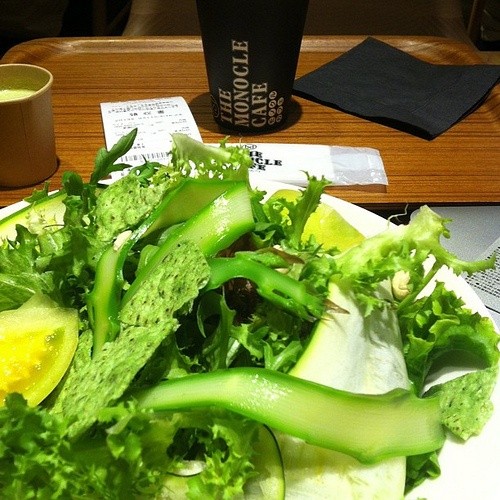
top-left (0, 175), bottom-right (499, 500)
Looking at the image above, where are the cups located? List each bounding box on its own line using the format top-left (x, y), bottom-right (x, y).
top-left (0, 64), bottom-right (57, 188)
top-left (196, 0), bottom-right (308, 133)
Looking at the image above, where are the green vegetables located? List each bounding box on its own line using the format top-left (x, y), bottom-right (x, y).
top-left (0, 130), bottom-right (500, 500)
